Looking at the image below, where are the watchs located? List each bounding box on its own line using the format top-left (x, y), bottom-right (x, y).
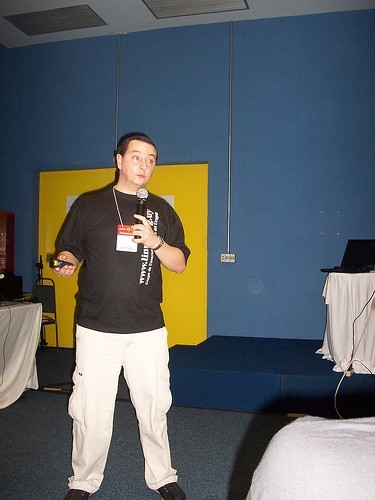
top-left (152, 236), bottom-right (164, 251)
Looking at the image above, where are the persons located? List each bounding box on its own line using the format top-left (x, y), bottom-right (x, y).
top-left (51, 134), bottom-right (192, 500)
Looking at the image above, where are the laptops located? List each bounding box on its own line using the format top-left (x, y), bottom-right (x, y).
top-left (320, 239), bottom-right (375, 274)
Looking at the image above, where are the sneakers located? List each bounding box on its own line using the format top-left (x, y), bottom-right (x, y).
top-left (154, 483), bottom-right (187, 500)
top-left (63, 489), bottom-right (90, 500)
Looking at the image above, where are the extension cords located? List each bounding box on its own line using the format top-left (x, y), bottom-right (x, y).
top-left (345, 368), bottom-right (353, 377)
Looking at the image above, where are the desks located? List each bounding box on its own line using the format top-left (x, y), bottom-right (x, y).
top-left (0, 302), bottom-right (43, 409)
top-left (315, 272), bottom-right (375, 374)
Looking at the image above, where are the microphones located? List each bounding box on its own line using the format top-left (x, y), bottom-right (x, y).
top-left (134, 188), bottom-right (148, 239)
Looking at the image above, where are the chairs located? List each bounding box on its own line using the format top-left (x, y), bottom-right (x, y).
top-left (32, 278), bottom-right (58, 352)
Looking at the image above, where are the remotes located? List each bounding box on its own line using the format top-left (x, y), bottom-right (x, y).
top-left (49, 257), bottom-right (74, 268)
